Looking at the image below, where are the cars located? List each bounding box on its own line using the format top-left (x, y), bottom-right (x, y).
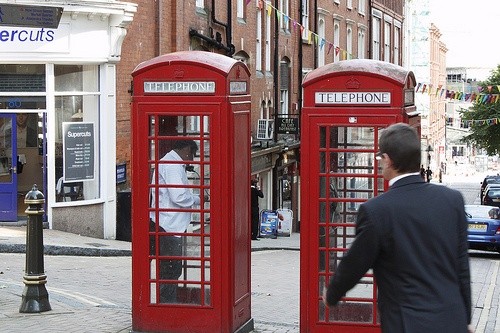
top-left (462, 204), bottom-right (500, 256)
top-left (481, 173), bottom-right (500, 204)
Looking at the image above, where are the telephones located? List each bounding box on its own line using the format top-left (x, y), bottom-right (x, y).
top-left (186, 166), bottom-right (194, 172)
top-left (320, 176), bottom-right (338, 224)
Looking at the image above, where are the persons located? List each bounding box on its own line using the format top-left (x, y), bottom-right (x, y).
top-left (425, 166), bottom-right (432, 181)
top-left (5, 104), bottom-right (36, 147)
top-left (420, 164), bottom-right (425, 182)
top-left (250, 179), bottom-right (264, 240)
top-left (321, 121), bottom-right (473, 333)
top-left (150, 138), bottom-right (209, 304)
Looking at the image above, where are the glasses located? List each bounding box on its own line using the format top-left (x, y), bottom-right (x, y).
top-left (375, 147), bottom-right (398, 160)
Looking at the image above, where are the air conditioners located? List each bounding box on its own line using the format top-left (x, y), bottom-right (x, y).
top-left (256, 119), bottom-right (274, 139)
